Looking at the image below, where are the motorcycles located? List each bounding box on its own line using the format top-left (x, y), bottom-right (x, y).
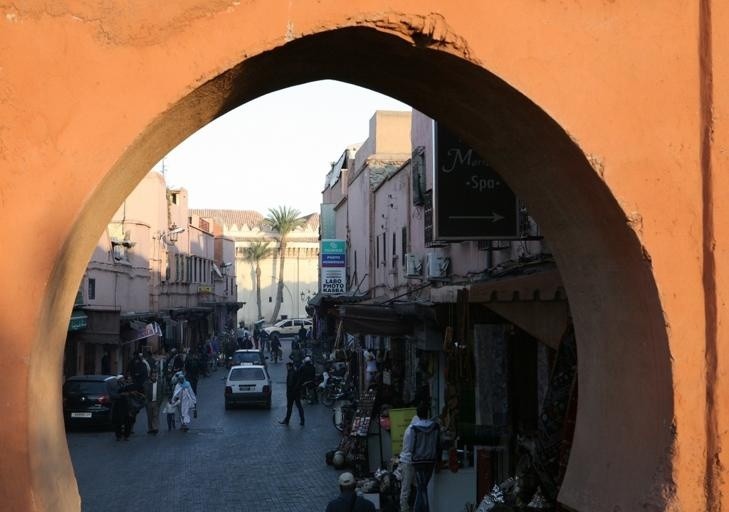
top-left (320, 377), bottom-right (359, 407)
top-left (294, 366), bottom-right (316, 406)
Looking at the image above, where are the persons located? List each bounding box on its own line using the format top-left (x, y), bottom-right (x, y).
top-left (109, 337), bottom-right (200, 441)
top-left (277, 363), bottom-right (306, 425)
top-left (299, 356), bottom-right (319, 404)
top-left (325, 471), bottom-right (377, 511)
top-left (289, 344), bottom-right (301, 362)
top-left (298, 324), bottom-right (307, 339)
top-left (396, 416), bottom-right (421, 512)
top-left (306, 326), bottom-right (313, 338)
top-left (200, 320), bottom-right (283, 375)
top-left (406, 402), bottom-right (439, 512)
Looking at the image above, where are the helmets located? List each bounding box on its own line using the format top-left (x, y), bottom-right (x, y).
top-left (305, 356), bottom-right (312, 364)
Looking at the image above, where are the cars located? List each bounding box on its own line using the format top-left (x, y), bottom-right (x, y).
top-left (227, 348), bottom-right (269, 372)
top-left (61, 372), bottom-right (130, 433)
top-left (260, 316), bottom-right (314, 340)
top-left (223, 361), bottom-right (274, 412)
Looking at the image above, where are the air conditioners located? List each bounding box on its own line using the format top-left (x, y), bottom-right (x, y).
top-left (403, 254), bottom-right (422, 275)
top-left (424, 251), bottom-right (451, 278)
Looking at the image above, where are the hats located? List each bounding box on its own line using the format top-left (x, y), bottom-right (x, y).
top-left (339, 473), bottom-right (354, 486)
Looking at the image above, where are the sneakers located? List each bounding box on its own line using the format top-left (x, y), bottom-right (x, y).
top-left (184, 425), bottom-right (189, 432)
top-left (148, 429), bottom-right (160, 435)
top-left (280, 421), bottom-right (304, 425)
